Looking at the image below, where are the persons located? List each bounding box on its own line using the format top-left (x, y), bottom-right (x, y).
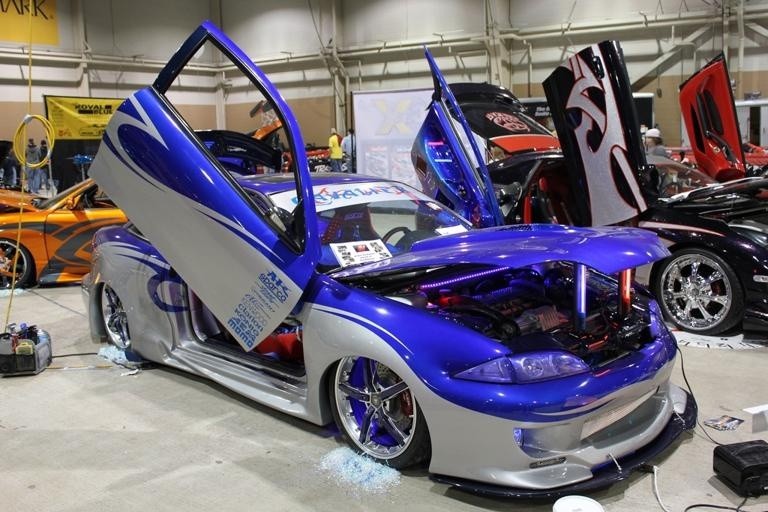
top-left (643, 127), bottom-right (674, 196)
top-left (677, 150), bottom-right (692, 190)
top-left (340, 128), bottom-right (356, 173)
top-left (665, 149), bottom-right (678, 193)
top-left (327, 127), bottom-right (343, 173)
top-left (1, 136), bottom-right (52, 195)
